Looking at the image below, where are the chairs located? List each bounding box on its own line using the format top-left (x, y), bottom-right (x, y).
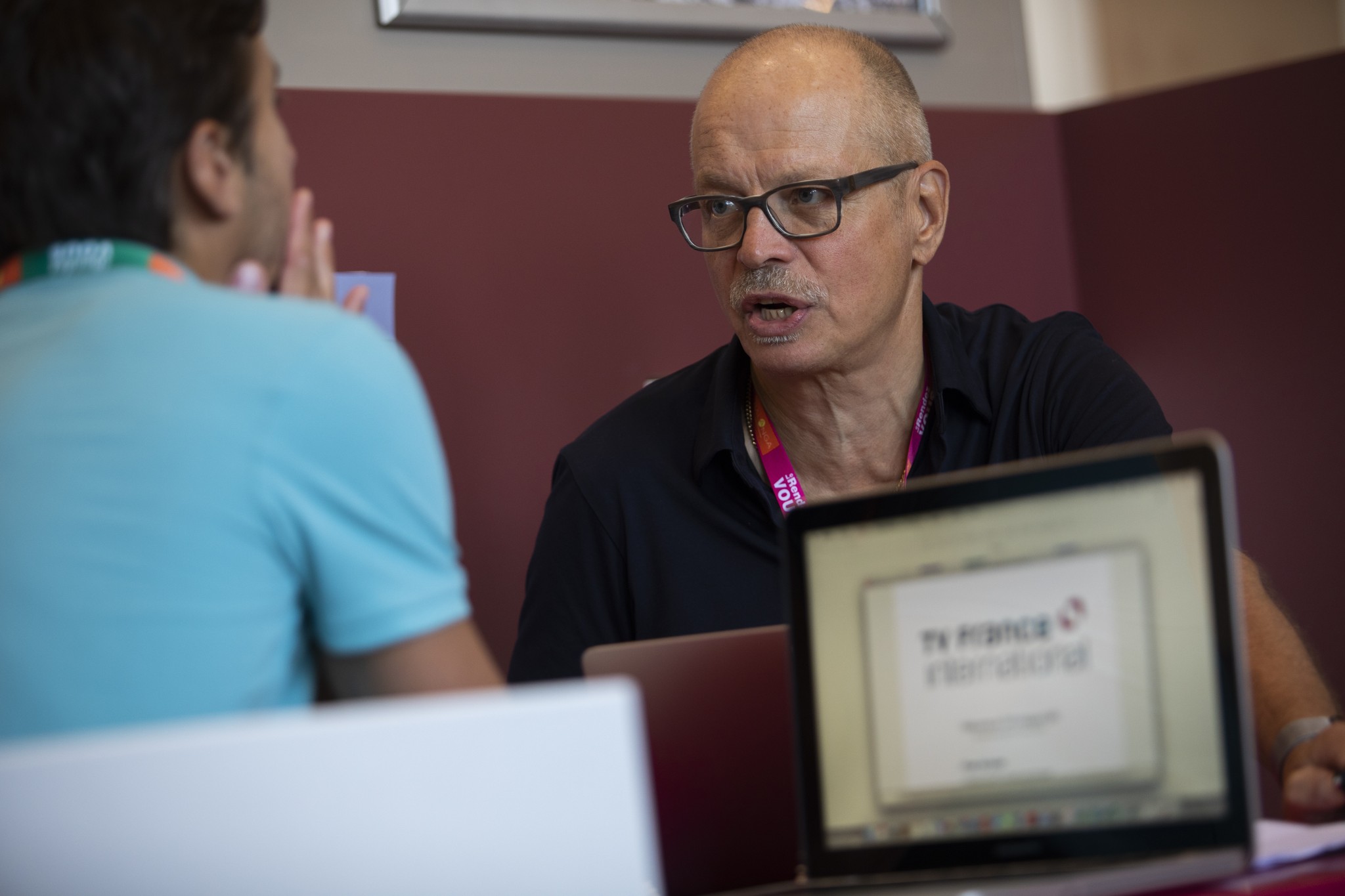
top-left (0, 669), bottom-right (667, 896)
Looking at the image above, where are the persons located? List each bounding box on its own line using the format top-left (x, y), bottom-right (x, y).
top-left (1, 0), bottom-right (508, 748)
top-left (518, 23), bottom-right (1345, 826)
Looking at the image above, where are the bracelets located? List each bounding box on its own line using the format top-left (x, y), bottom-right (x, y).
top-left (1269, 715), bottom-right (1345, 787)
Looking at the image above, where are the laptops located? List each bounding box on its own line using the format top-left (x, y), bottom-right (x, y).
top-left (580, 627), bottom-right (788, 895)
top-left (753, 424), bottom-right (1262, 894)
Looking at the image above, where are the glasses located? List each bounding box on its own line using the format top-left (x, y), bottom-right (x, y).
top-left (668, 162), bottom-right (918, 253)
